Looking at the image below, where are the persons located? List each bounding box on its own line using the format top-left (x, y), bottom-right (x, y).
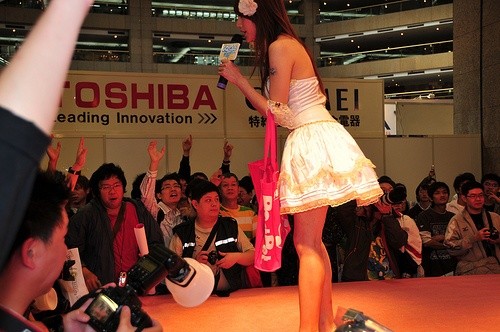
top-left (321, 170), bottom-right (500, 281)
top-left (0, 0), bottom-right (95, 268)
top-left (0, 135), bottom-right (301, 332)
top-left (218, 0), bottom-right (383, 332)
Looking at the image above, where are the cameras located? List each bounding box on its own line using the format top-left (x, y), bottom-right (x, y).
top-left (206, 250), bottom-right (223, 265)
top-left (378, 184), bottom-right (407, 207)
top-left (334, 310), bottom-right (376, 332)
top-left (85, 241), bottom-right (196, 332)
top-left (484, 228), bottom-right (499, 240)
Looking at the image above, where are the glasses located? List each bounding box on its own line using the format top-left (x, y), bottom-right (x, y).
top-left (100, 183), bottom-right (123, 191)
top-left (161, 184), bottom-right (181, 191)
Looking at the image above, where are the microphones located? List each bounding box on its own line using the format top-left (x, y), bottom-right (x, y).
top-left (217, 33), bottom-right (243, 89)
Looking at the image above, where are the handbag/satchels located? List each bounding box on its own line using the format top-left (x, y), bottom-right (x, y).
top-left (247, 108), bottom-right (291, 272)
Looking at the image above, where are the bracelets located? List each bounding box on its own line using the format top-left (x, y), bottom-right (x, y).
top-left (223, 160), bottom-right (230, 163)
top-left (68, 167), bottom-right (81, 175)
